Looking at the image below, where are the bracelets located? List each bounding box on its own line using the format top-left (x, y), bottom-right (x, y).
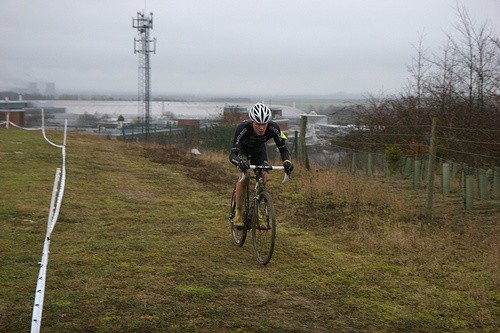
top-left (284, 160), bottom-right (291, 164)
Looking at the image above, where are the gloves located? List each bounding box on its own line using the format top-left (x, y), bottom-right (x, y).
top-left (284, 159), bottom-right (294, 175)
top-left (231, 157), bottom-right (250, 173)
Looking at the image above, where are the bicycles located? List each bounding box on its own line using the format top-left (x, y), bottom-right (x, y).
top-left (229, 154), bottom-right (289, 266)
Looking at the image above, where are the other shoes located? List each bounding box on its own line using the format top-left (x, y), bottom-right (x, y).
top-left (258, 218), bottom-right (269, 229)
top-left (234, 214), bottom-right (244, 226)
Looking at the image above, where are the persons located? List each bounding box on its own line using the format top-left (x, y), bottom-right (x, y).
top-left (229, 102), bottom-right (294, 229)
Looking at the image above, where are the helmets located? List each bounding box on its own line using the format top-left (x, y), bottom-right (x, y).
top-left (249, 103), bottom-right (272, 124)
